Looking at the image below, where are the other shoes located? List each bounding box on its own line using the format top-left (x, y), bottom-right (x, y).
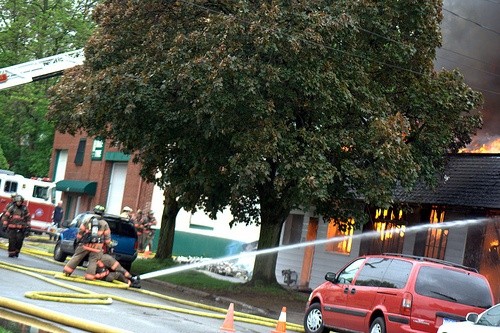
top-left (9, 253), bottom-right (19, 258)
top-left (63, 270), bottom-right (70, 277)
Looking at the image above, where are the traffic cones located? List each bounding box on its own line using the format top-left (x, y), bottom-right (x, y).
top-left (270, 306), bottom-right (287, 333)
top-left (218, 303), bottom-right (236, 333)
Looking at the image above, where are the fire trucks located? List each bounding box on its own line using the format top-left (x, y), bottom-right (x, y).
top-left (0, 169), bottom-right (57, 239)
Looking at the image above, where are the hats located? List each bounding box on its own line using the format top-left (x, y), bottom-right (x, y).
top-left (123, 206), bottom-right (132, 211)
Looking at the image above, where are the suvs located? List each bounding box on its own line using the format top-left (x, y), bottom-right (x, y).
top-left (54, 211), bottom-right (139, 273)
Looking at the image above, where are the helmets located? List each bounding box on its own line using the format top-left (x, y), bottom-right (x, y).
top-left (14, 195), bottom-right (23, 202)
top-left (94, 205), bottom-right (105, 214)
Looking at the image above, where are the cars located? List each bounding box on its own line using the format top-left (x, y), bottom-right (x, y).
top-left (436, 304), bottom-right (500, 332)
top-left (303, 252), bottom-right (495, 333)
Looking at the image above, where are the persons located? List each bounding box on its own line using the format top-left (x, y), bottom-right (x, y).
top-left (95, 254), bottom-right (142, 289)
top-left (120, 206), bottom-right (157, 255)
top-left (63, 204), bottom-right (111, 281)
top-left (49, 200), bottom-right (64, 241)
top-left (0, 193), bottom-right (31, 258)
